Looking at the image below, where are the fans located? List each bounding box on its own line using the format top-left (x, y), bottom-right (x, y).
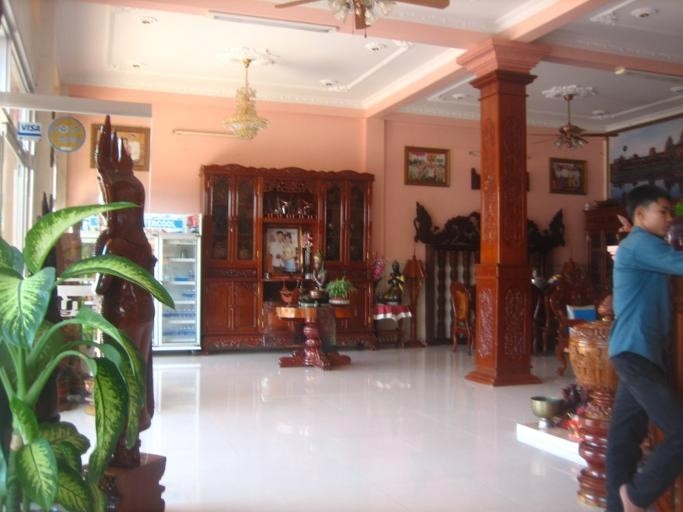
top-left (527, 94), bottom-right (619, 145)
top-left (272, 1), bottom-right (452, 31)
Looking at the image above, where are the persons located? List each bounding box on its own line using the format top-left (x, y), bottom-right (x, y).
top-left (600, 182), bottom-right (682, 512)
top-left (269, 230), bottom-right (284, 273)
top-left (122, 132), bottom-right (141, 165)
top-left (280, 232), bottom-right (297, 274)
top-left (554, 162), bottom-right (582, 190)
top-left (408, 154), bottom-right (448, 185)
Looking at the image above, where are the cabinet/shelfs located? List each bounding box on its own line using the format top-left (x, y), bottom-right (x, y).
top-left (582, 209), bottom-right (626, 303)
top-left (197, 163), bottom-right (374, 355)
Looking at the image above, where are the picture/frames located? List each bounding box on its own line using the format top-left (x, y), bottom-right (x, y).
top-left (548, 156), bottom-right (588, 196)
top-left (404, 146), bottom-right (450, 188)
top-left (89, 123), bottom-right (150, 172)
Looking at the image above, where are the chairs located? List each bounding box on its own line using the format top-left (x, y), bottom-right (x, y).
top-left (449, 281), bottom-right (476, 357)
top-left (530, 272), bottom-right (601, 375)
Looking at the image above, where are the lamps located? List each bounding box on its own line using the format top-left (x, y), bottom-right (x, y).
top-left (325, 1), bottom-right (398, 32)
top-left (223, 57), bottom-right (270, 142)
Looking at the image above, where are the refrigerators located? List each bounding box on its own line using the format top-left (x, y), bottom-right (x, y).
top-left (80, 213), bottom-right (202, 354)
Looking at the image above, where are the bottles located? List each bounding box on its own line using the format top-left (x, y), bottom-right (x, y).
top-left (179, 247), bottom-right (188, 258)
top-left (160, 269), bottom-right (196, 343)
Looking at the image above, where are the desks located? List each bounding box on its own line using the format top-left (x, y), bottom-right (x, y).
top-left (273, 306), bottom-right (350, 371)
top-left (374, 303), bottom-right (408, 349)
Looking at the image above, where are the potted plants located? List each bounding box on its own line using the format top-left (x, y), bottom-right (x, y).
top-left (325, 271), bottom-right (357, 306)
